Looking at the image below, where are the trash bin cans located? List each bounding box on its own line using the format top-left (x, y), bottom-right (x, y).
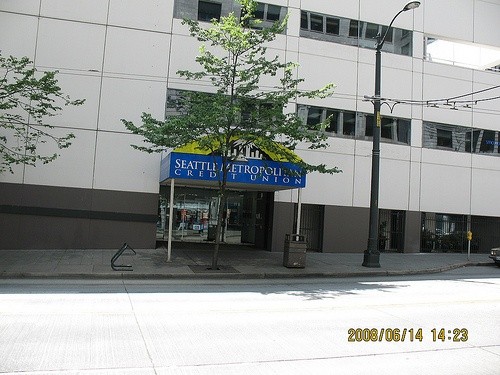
top-left (283, 232), bottom-right (308, 268)
top-left (208, 224), bottom-right (218, 241)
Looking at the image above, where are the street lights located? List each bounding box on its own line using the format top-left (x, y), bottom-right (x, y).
top-left (361, 0), bottom-right (421, 268)
